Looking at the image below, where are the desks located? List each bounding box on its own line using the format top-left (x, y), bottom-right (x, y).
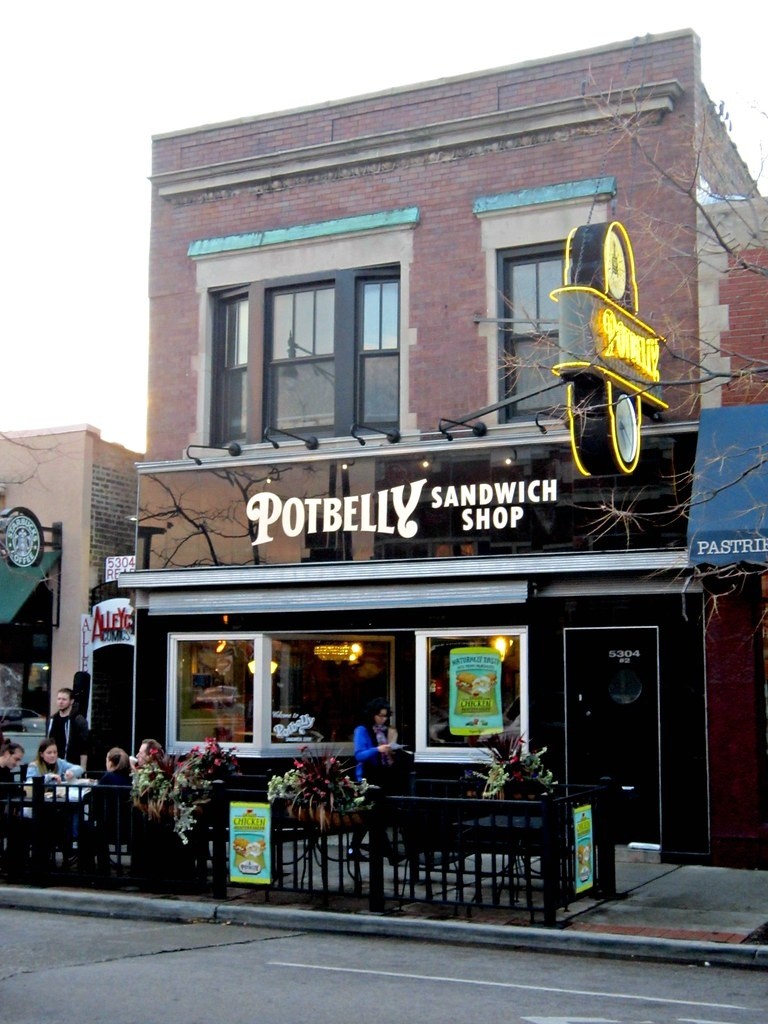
top-left (454, 817), bottom-right (542, 904)
top-left (0, 796), bottom-right (80, 868)
top-left (180, 702), bottom-right (245, 741)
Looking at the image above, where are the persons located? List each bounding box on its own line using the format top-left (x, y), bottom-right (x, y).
top-left (347, 697), bottom-right (408, 866)
top-left (0, 685), bottom-right (165, 875)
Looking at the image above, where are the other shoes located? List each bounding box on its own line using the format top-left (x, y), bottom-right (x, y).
top-left (389, 854), bottom-right (406, 865)
top-left (346, 847), bottom-right (369, 862)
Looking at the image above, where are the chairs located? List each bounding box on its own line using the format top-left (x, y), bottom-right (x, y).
top-left (227, 769), bottom-right (281, 886)
top-left (13, 765), bottom-right (107, 860)
top-left (410, 778), bottom-right (463, 899)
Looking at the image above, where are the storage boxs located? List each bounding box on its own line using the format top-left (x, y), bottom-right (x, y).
top-left (498, 778), bottom-right (541, 802)
top-left (318, 803), bottom-right (359, 827)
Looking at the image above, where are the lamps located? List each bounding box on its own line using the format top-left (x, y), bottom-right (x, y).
top-left (437, 417), bottom-right (486, 442)
top-left (349, 424), bottom-right (400, 446)
top-left (535, 411), bottom-right (569, 434)
top-left (186, 443), bottom-right (240, 465)
top-left (263, 426), bottom-right (318, 450)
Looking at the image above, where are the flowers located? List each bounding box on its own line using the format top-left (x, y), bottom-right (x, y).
top-left (474, 735), bottom-right (559, 800)
top-left (131, 738), bottom-right (241, 847)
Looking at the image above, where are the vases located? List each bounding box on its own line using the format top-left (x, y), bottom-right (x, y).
top-left (309, 801), bottom-right (320, 821)
top-left (133, 795), bottom-right (173, 818)
top-left (268, 733), bottom-right (379, 827)
top-left (298, 805), bottom-right (307, 821)
top-left (286, 798), bottom-right (298, 822)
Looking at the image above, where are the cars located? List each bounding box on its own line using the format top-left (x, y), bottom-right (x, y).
top-left (193, 684), bottom-right (240, 707)
top-left (0, 707), bottom-right (47, 733)
top-left (428, 693), bottom-right (521, 748)
